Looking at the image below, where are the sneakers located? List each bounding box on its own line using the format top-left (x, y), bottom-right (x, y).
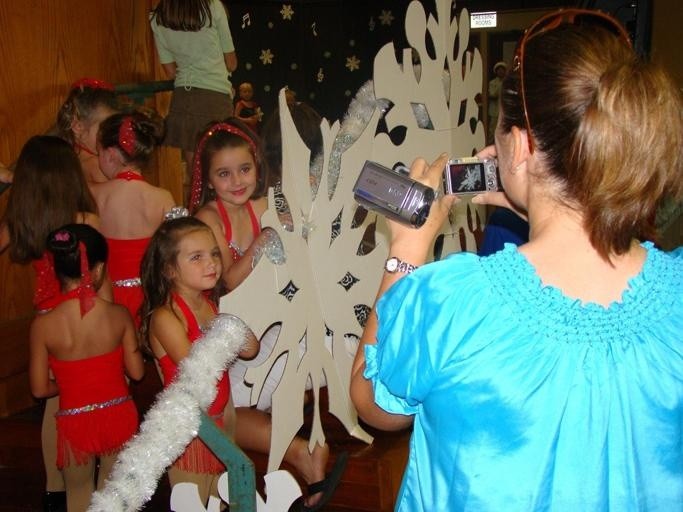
top-left (43, 491), bottom-right (66, 512)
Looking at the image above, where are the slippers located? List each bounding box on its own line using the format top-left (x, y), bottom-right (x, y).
top-left (299, 452), bottom-right (348, 512)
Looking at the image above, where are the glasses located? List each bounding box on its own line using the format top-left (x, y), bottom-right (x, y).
top-left (516, 9), bottom-right (632, 155)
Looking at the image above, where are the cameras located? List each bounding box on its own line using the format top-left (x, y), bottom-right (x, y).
top-left (352, 160), bottom-right (435, 229)
top-left (446, 156), bottom-right (500, 198)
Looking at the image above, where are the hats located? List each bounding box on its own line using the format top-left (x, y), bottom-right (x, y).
top-left (493, 62), bottom-right (507, 74)
